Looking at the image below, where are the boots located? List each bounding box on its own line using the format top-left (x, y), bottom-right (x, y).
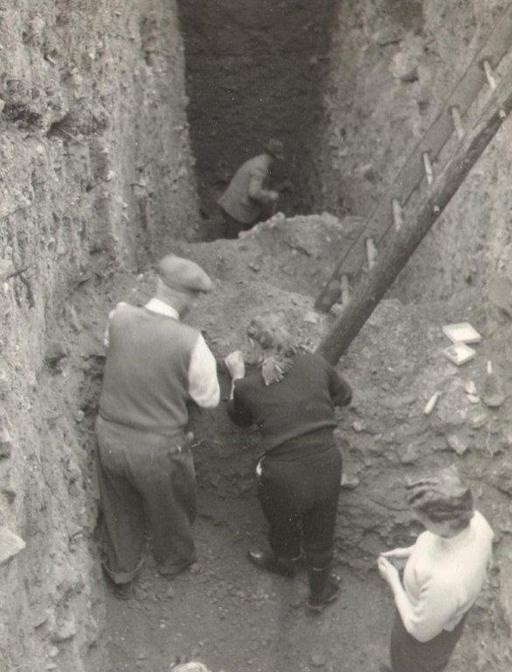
top-left (306, 562), bottom-right (341, 612)
top-left (248, 545), bottom-right (305, 579)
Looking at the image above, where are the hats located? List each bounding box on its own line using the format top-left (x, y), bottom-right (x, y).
top-left (158, 253), bottom-right (213, 297)
top-left (262, 138), bottom-right (285, 161)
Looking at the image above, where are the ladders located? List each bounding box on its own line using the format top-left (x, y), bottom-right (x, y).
top-left (313, 4), bottom-right (512, 368)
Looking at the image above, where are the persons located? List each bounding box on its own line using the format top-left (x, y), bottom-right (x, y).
top-left (224, 313), bottom-right (354, 613)
top-left (215, 136), bottom-right (287, 241)
top-left (375, 465), bottom-right (496, 672)
top-left (93, 251), bottom-right (221, 602)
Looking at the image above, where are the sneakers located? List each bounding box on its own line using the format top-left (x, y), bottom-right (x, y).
top-left (115, 580), bottom-right (132, 600)
